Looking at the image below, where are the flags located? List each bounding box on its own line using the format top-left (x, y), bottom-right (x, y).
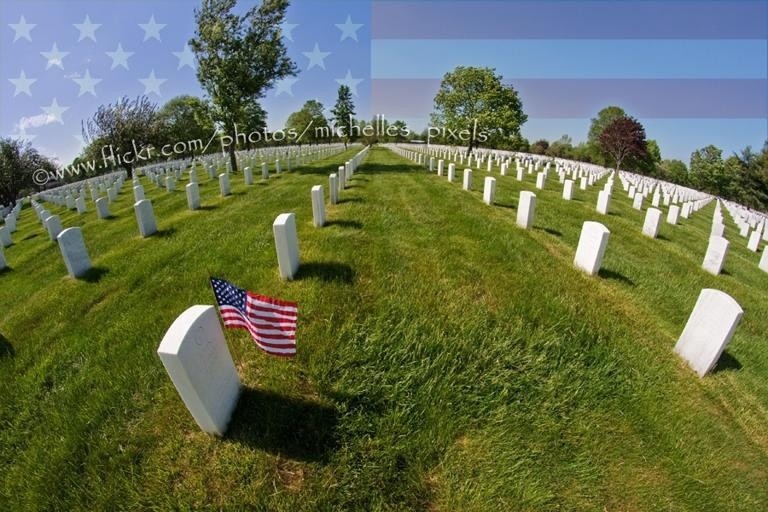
top-left (210, 278), bottom-right (298, 356)
